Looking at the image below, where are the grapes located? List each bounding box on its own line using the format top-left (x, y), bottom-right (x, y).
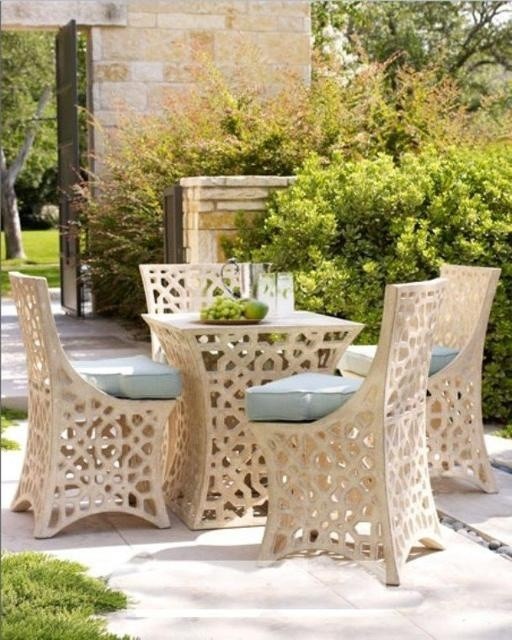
top-left (201, 294), bottom-right (245, 321)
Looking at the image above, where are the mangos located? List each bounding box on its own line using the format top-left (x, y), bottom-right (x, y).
top-left (240, 300), bottom-right (268, 319)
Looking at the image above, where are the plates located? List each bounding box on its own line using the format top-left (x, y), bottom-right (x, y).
top-left (202, 318), bottom-right (263, 324)
